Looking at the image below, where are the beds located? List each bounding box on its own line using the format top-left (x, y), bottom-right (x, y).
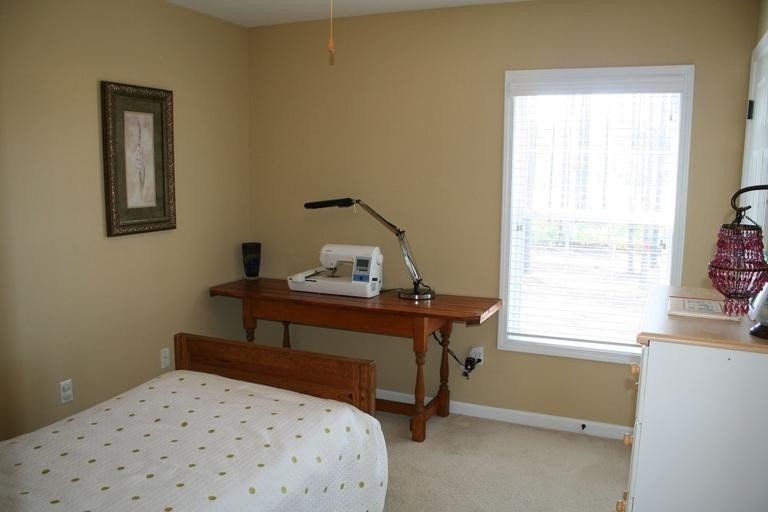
top-left (1, 329), bottom-right (392, 512)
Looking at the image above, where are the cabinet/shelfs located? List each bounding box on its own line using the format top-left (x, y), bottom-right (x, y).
top-left (618, 284), bottom-right (767, 512)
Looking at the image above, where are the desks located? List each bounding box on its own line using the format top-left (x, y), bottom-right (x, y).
top-left (207, 276), bottom-right (505, 445)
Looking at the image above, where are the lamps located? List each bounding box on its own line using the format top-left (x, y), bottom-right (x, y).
top-left (303, 196), bottom-right (436, 302)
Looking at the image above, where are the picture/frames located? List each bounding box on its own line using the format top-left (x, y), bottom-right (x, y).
top-left (99, 77), bottom-right (179, 238)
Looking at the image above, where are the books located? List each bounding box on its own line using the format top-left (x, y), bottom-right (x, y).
top-left (668, 288), bottom-right (739, 321)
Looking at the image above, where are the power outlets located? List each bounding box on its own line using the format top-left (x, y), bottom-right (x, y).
top-left (466, 344), bottom-right (485, 369)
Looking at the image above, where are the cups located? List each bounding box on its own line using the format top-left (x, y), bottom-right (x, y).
top-left (241, 241), bottom-right (262, 281)
